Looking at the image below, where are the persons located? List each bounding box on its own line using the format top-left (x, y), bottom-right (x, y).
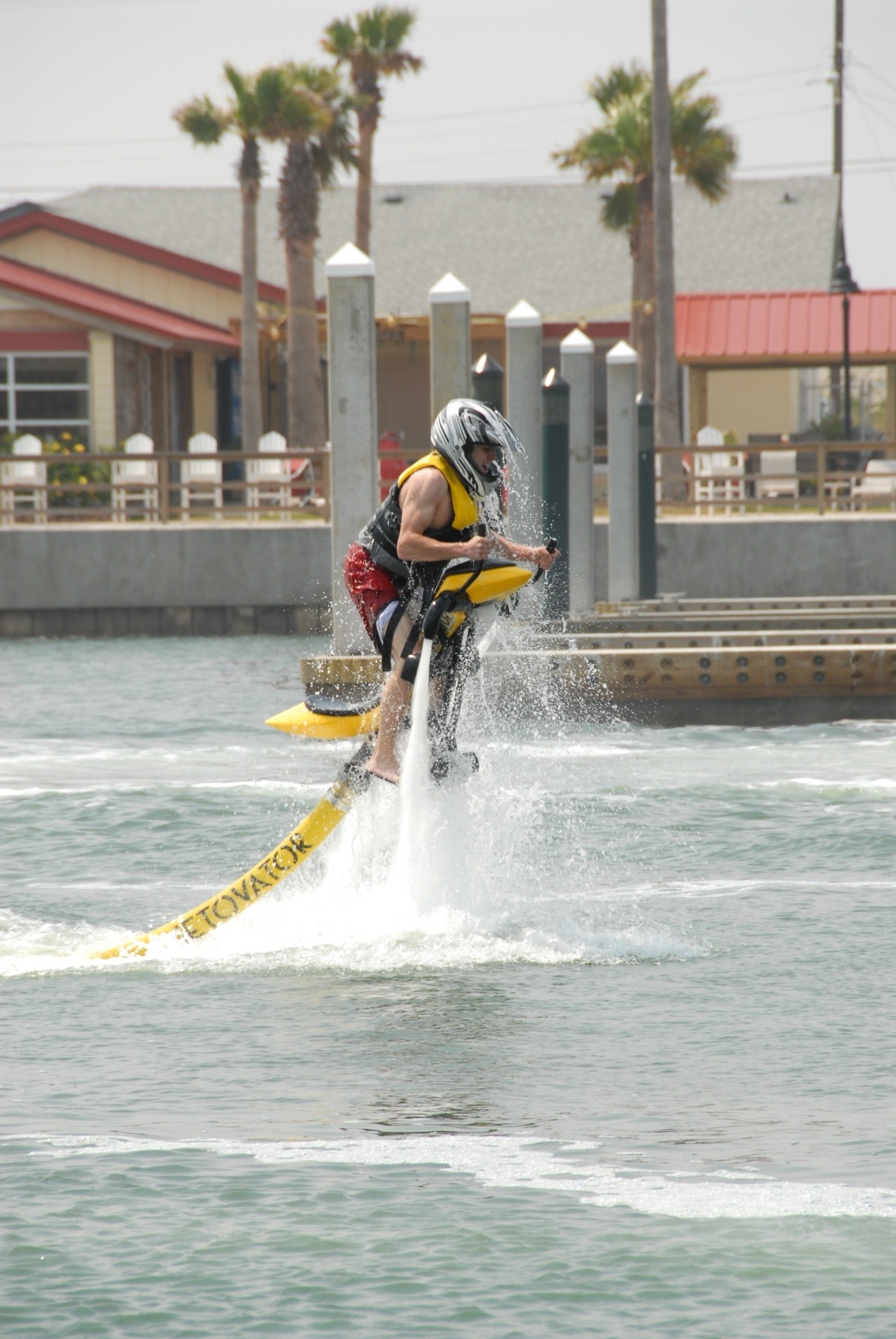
top-left (343, 398), bottom-right (560, 785)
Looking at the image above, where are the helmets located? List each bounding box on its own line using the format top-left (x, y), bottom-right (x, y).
top-left (430, 397), bottom-right (508, 496)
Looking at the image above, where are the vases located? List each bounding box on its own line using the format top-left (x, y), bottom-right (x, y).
top-left (53, 498), bottom-right (81, 520)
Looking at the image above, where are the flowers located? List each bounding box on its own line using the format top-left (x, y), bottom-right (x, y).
top-left (48, 430), bottom-right (91, 497)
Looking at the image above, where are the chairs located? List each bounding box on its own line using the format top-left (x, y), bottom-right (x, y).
top-left (694, 426), bottom-right (746, 515)
top-left (0, 434), bottom-right (48, 524)
top-left (754, 450), bottom-right (800, 512)
top-left (180, 431), bottom-right (222, 521)
top-left (849, 459), bottom-right (896, 514)
top-left (244, 430), bottom-right (291, 520)
top-left (111, 432), bottom-right (161, 522)
